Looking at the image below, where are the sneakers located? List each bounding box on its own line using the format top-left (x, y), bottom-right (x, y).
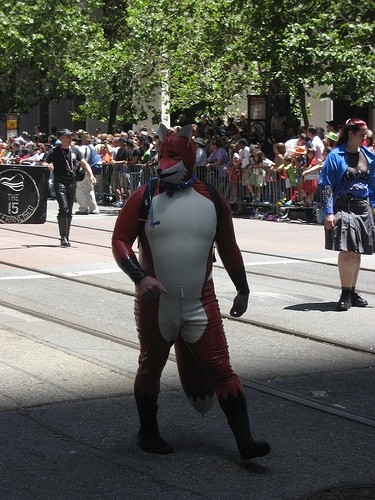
top-left (351, 291), bottom-right (368, 307)
top-left (337, 289), bottom-right (353, 311)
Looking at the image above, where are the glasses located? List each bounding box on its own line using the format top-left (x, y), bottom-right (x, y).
top-left (61, 135), bottom-right (74, 139)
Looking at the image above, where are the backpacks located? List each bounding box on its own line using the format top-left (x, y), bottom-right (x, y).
top-left (86, 145), bottom-right (103, 175)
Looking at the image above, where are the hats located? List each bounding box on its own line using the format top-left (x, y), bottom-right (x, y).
top-left (118, 137), bottom-right (127, 143)
top-left (194, 138), bottom-right (206, 146)
top-left (77, 134), bottom-right (94, 142)
top-left (316, 127), bottom-right (324, 134)
top-left (325, 121), bottom-right (337, 127)
top-left (294, 146), bottom-right (308, 155)
top-left (325, 131), bottom-right (340, 142)
top-left (57, 128), bottom-right (73, 139)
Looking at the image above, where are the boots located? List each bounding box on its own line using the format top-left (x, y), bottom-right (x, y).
top-left (67, 214), bottom-right (72, 247)
top-left (58, 216), bottom-right (69, 246)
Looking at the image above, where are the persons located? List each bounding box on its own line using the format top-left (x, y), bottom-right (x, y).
top-left (111, 133), bottom-right (273, 462)
top-left (316, 117), bottom-right (375, 310)
top-left (41, 127), bottom-right (97, 248)
top-left (0, 114), bottom-right (375, 222)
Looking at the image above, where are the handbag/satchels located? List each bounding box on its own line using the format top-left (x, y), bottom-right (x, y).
top-left (75, 162), bottom-right (86, 181)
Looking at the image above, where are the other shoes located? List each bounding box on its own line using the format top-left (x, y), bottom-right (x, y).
top-left (90, 210), bottom-right (99, 214)
top-left (96, 199), bottom-right (125, 207)
top-left (75, 210), bottom-right (89, 215)
top-left (223, 194), bottom-right (315, 216)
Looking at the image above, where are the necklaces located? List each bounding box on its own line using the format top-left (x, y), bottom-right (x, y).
top-left (62, 148), bottom-right (71, 158)
top-left (59, 144), bottom-right (74, 174)
top-left (159, 175), bottom-right (196, 192)
